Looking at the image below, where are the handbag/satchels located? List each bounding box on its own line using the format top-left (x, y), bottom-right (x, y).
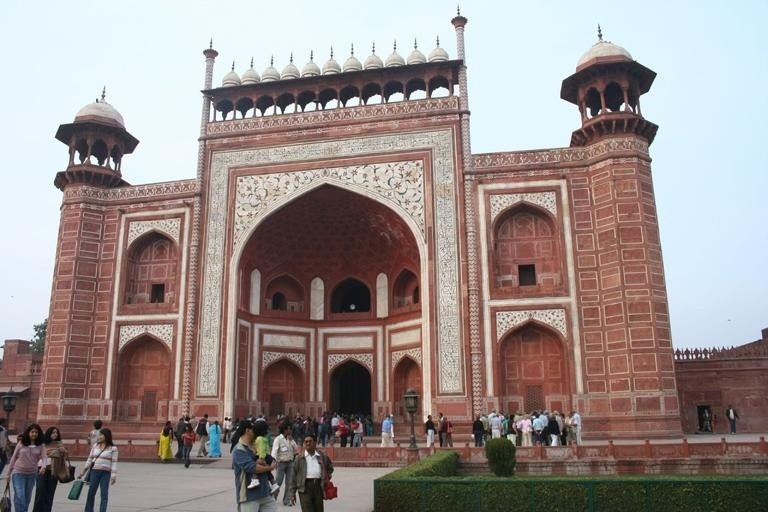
top-left (0, 483), bottom-right (11, 512)
top-left (68, 481), bottom-right (84, 500)
top-left (58, 455), bottom-right (75, 484)
top-left (80, 463), bottom-right (94, 482)
top-left (322, 479), bottom-right (336, 500)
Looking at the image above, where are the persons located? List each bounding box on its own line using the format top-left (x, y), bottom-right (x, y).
top-left (246, 421), bottom-right (280, 494)
top-left (725, 405), bottom-right (740, 434)
top-left (289, 435), bottom-right (334, 512)
top-left (702, 409), bottom-right (711, 432)
top-left (473, 409), bottom-right (582, 447)
top-left (271, 423), bottom-right (298, 506)
top-left (424, 411), bottom-right (455, 448)
top-left (87, 420), bottom-right (103, 453)
top-left (158, 411), bottom-right (396, 468)
top-left (6, 424), bottom-right (47, 512)
top-left (0, 417), bottom-right (30, 474)
top-left (76, 428), bottom-right (118, 512)
top-left (231, 420), bottom-right (278, 512)
top-left (32, 426), bottom-right (68, 512)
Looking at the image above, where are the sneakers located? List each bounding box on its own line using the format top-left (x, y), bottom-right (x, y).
top-left (247, 479), bottom-right (259, 489)
top-left (270, 484), bottom-right (278, 494)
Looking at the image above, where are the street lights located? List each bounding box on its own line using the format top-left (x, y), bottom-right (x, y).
top-left (403, 387), bottom-right (420, 449)
top-left (2, 385), bottom-right (17, 432)
